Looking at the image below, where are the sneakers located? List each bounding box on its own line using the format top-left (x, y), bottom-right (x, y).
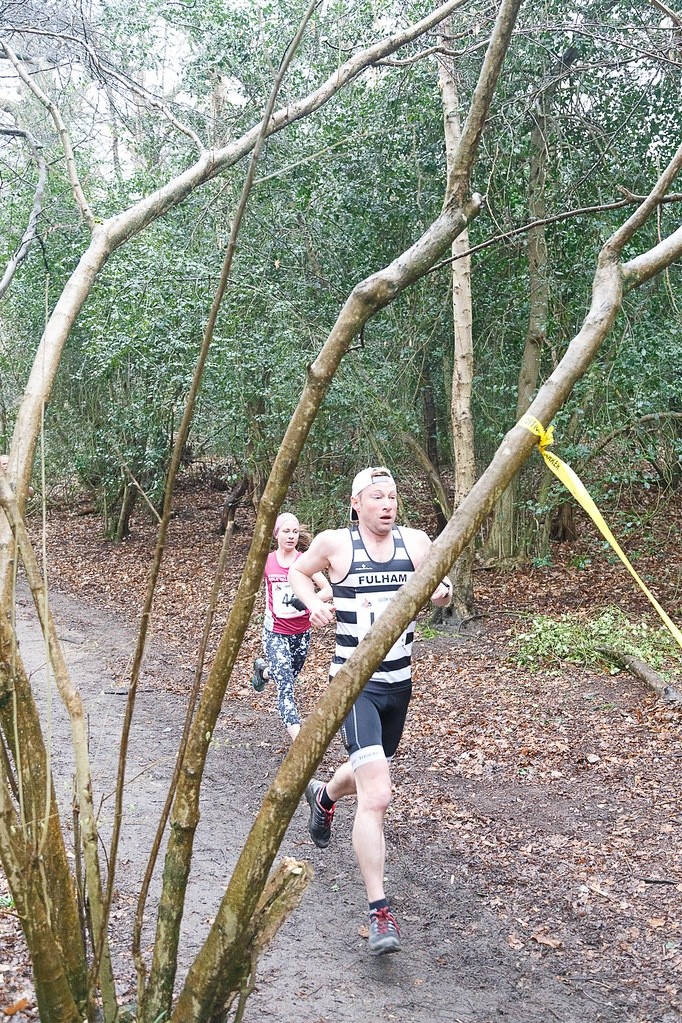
top-left (305, 777), bottom-right (337, 848)
top-left (367, 905), bottom-right (403, 957)
top-left (253, 657), bottom-right (270, 693)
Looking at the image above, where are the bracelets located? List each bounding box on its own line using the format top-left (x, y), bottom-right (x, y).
top-left (441, 579), bottom-right (450, 599)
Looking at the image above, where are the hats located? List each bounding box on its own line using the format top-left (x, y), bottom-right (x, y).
top-left (349, 466), bottom-right (396, 522)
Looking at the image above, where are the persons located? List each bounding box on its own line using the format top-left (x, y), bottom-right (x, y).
top-left (253, 512), bottom-right (333, 741)
top-left (0, 455), bottom-right (34, 497)
top-left (286, 466), bottom-right (455, 958)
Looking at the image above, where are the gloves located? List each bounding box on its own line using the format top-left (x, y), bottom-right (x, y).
top-left (286, 598), bottom-right (308, 611)
